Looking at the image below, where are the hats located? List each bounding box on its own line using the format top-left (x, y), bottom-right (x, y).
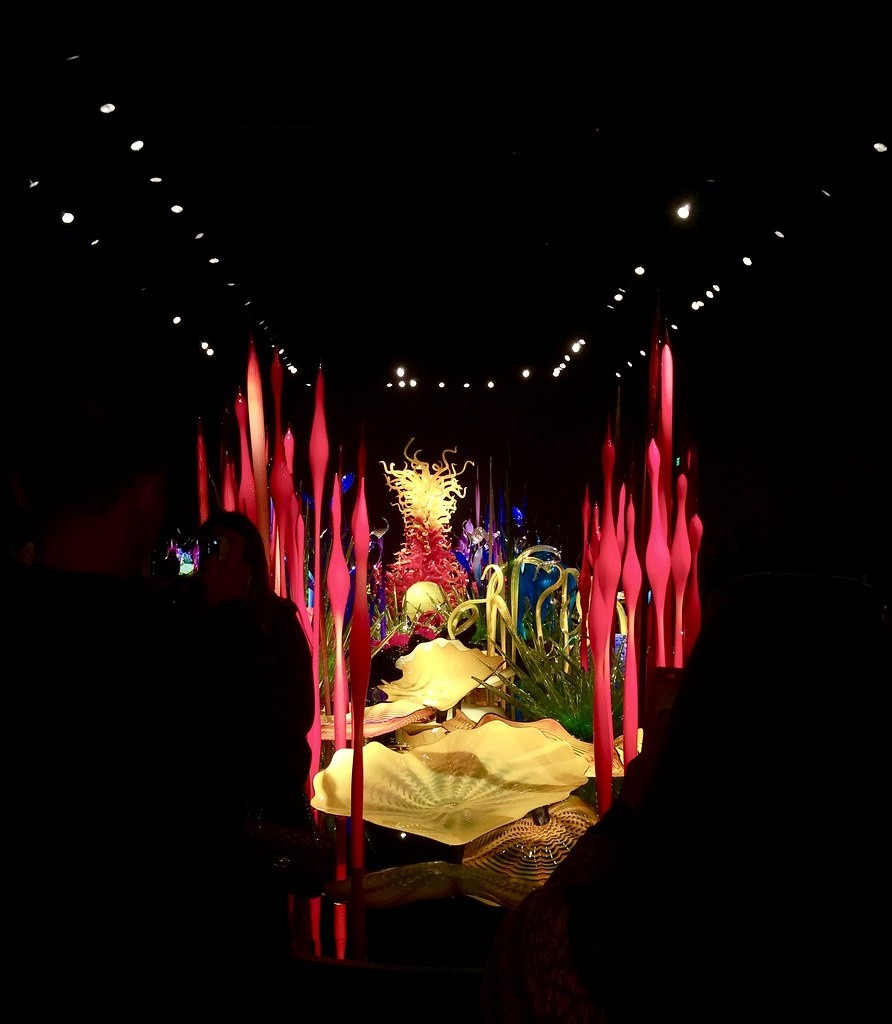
top-left (201, 512), bottom-right (259, 546)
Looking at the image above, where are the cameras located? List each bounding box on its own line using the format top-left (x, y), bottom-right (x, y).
top-left (166, 536), bottom-right (228, 579)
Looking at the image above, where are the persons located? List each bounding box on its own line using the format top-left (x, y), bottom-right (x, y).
top-left (0, 378), bottom-right (263, 973)
top-left (197, 512), bottom-right (314, 955)
